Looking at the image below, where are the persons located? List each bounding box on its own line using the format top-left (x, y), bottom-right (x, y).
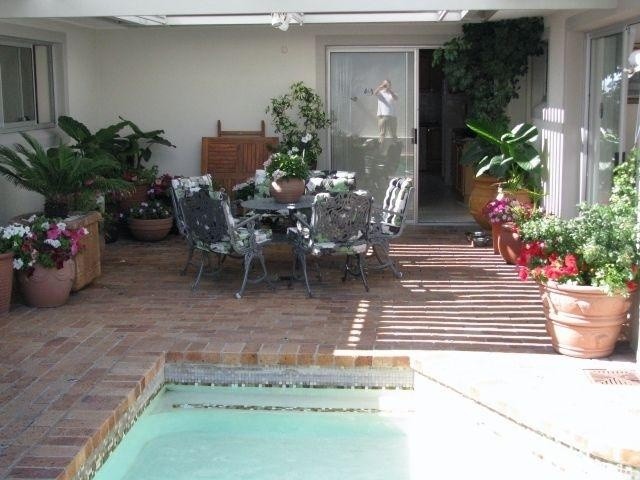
top-left (373, 79), bottom-right (398, 145)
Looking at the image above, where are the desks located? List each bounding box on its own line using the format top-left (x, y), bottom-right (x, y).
top-left (199, 120), bottom-right (280, 190)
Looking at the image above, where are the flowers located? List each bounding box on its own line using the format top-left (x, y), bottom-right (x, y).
top-left (426, 19), bottom-right (639, 362)
top-left (262, 150), bottom-right (312, 182)
top-left (1, 110), bottom-right (183, 312)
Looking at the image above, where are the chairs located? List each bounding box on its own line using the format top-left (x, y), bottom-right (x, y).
top-left (166, 169), bottom-right (416, 298)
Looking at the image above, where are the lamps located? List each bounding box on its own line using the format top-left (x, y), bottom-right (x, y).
top-left (268, 11), bottom-right (308, 34)
